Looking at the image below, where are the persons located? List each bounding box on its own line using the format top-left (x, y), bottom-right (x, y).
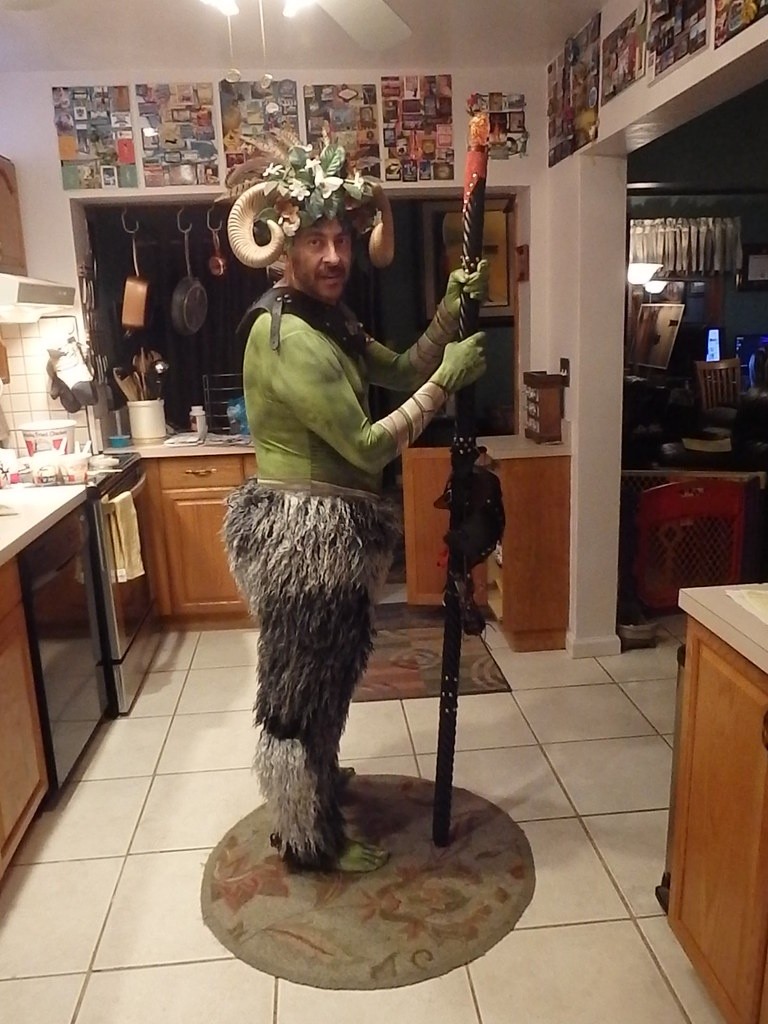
top-left (222, 186), bottom-right (491, 872)
top-left (731, 347), bottom-right (768, 465)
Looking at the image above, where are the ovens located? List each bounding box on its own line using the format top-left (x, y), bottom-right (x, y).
top-left (85, 462), bottom-right (164, 713)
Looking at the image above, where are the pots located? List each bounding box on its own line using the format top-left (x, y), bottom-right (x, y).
top-left (121, 231), bottom-right (151, 337)
top-left (171, 230), bottom-right (208, 335)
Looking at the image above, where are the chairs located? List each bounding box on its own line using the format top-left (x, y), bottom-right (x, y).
top-left (693, 358), bottom-right (742, 413)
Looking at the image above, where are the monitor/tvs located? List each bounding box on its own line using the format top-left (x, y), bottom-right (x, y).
top-left (735, 334), bottom-right (768, 375)
top-left (707, 327), bottom-right (720, 363)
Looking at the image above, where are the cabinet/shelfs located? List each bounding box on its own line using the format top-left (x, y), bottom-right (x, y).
top-left (202, 371), bottom-right (243, 434)
top-left (523, 371), bottom-right (564, 443)
top-left (140, 458), bottom-right (257, 627)
top-left (402, 435), bottom-right (572, 654)
top-left (667, 614), bottom-right (768, 1024)
top-left (0, 557), bottom-right (48, 881)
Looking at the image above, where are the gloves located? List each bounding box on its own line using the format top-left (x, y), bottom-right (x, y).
top-left (443, 258), bottom-right (490, 320)
top-left (427, 329), bottom-right (487, 396)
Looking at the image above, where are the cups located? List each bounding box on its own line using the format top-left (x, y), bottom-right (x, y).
top-left (31, 456), bottom-right (58, 486)
top-left (20, 420), bottom-right (77, 460)
top-left (59, 453), bottom-right (91, 483)
top-left (127, 399), bottom-right (166, 439)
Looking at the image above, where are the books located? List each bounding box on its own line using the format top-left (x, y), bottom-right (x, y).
top-left (164, 432), bottom-right (253, 448)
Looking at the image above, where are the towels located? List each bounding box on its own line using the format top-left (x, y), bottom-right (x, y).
top-left (108, 490), bottom-right (144, 584)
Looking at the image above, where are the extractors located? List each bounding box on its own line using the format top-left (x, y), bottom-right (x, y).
top-left (0, 273), bottom-right (75, 323)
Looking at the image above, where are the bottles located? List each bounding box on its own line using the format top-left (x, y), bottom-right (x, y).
top-left (190, 406), bottom-right (205, 432)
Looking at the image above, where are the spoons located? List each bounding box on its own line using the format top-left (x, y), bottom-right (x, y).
top-left (88, 469), bottom-right (122, 475)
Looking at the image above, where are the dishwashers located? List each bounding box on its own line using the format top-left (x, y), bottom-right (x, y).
top-left (16, 507), bottom-right (110, 788)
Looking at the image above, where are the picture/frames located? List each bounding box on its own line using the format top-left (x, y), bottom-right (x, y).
top-left (629, 303), bottom-right (686, 370)
top-left (415, 194), bottom-right (515, 330)
top-left (737, 240), bottom-right (768, 292)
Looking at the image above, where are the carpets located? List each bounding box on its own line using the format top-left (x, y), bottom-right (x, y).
top-left (351, 603), bottom-right (513, 706)
top-left (199, 773), bottom-right (537, 991)
